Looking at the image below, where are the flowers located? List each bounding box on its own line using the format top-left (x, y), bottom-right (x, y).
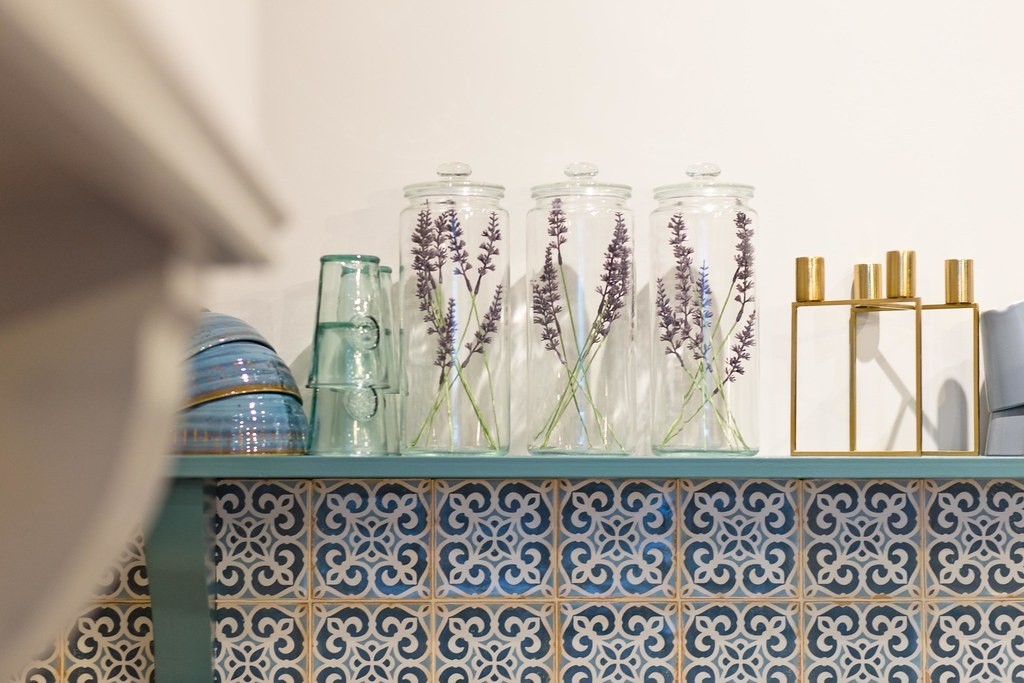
top-left (412, 200), bottom-right (757, 453)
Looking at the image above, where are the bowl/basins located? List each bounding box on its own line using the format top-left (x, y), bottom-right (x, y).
top-left (172, 307), bottom-right (310, 454)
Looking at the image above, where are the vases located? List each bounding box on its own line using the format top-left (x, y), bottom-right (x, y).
top-left (398, 161), bottom-right (509, 453)
top-left (524, 165), bottom-right (637, 453)
top-left (644, 164), bottom-right (765, 456)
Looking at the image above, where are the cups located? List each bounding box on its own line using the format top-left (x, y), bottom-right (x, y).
top-left (305, 253), bottom-right (401, 457)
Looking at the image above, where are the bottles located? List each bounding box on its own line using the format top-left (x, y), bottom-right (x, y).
top-left (648, 162), bottom-right (762, 456)
top-left (525, 163), bottom-right (640, 456)
top-left (397, 161), bottom-right (512, 457)
